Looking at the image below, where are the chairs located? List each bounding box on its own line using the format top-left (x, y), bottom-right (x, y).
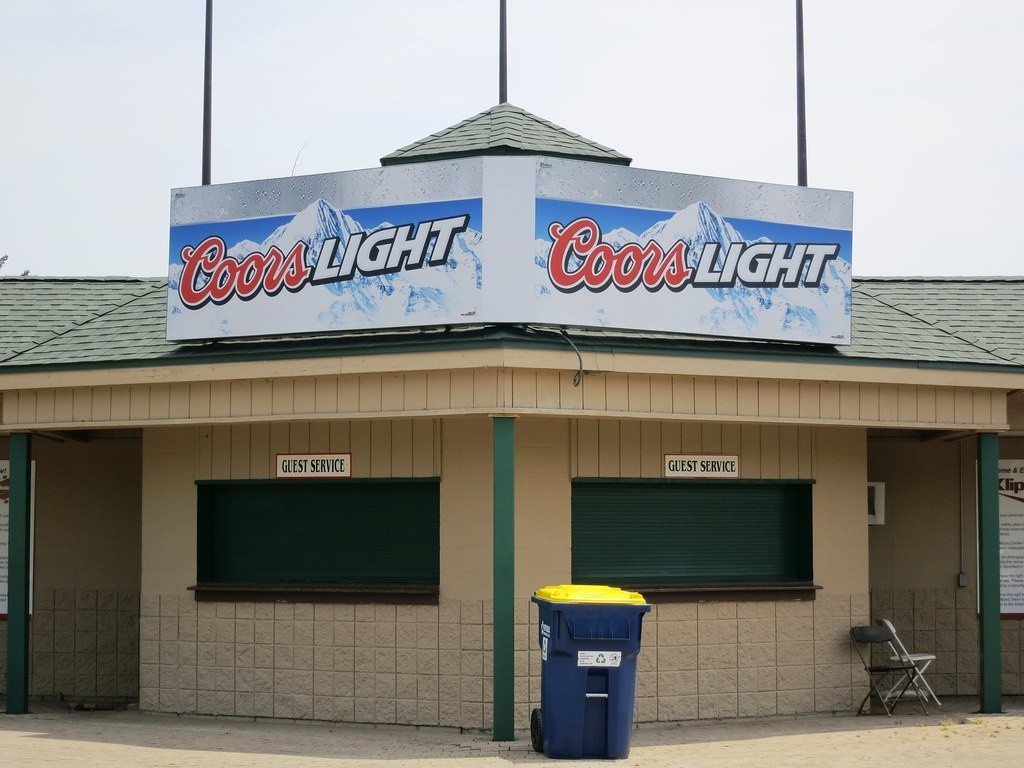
top-left (849, 625), bottom-right (930, 718)
top-left (875, 617), bottom-right (943, 706)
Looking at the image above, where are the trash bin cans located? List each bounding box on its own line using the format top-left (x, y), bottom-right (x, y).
top-left (531, 583), bottom-right (652, 760)
